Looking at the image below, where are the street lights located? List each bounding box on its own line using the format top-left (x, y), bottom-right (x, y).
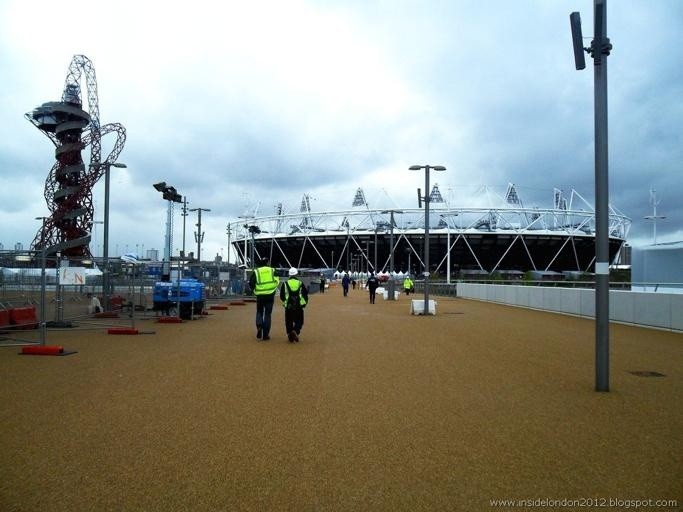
top-left (153, 181), bottom-right (182, 276)
top-left (239, 215), bottom-right (261, 269)
top-left (352, 229), bottom-right (384, 290)
top-left (409, 165), bottom-right (447, 316)
top-left (381, 210), bottom-right (403, 300)
top-left (189, 208), bottom-right (210, 263)
top-left (88, 162), bottom-right (127, 312)
top-left (35, 216), bottom-right (53, 249)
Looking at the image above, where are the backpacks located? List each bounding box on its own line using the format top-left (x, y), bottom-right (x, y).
top-left (285, 280), bottom-right (303, 310)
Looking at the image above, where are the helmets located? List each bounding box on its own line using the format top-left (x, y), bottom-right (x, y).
top-left (288, 267), bottom-right (299, 276)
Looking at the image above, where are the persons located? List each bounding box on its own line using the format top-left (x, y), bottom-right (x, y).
top-left (279, 266), bottom-right (308, 343)
top-left (351, 279), bottom-right (356, 290)
top-left (341, 273), bottom-right (350, 296)
top-left (403, 275), bottom-right (414, 297)
top-left (248, 257), bottom-right (280, 340)
top-left (206, 279), bottom-right (254, 296)
top-left (86, 292), bottom-right (100, 313)
top-left (319, 273), bottom-right (326, 294)
top-left (363, 272), bottom-right (379, 304)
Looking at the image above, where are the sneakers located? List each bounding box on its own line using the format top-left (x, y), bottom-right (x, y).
top-left (256, 327), bottom-right (270, 340)
top-left (288, 331), bottom-right (300, 344)
